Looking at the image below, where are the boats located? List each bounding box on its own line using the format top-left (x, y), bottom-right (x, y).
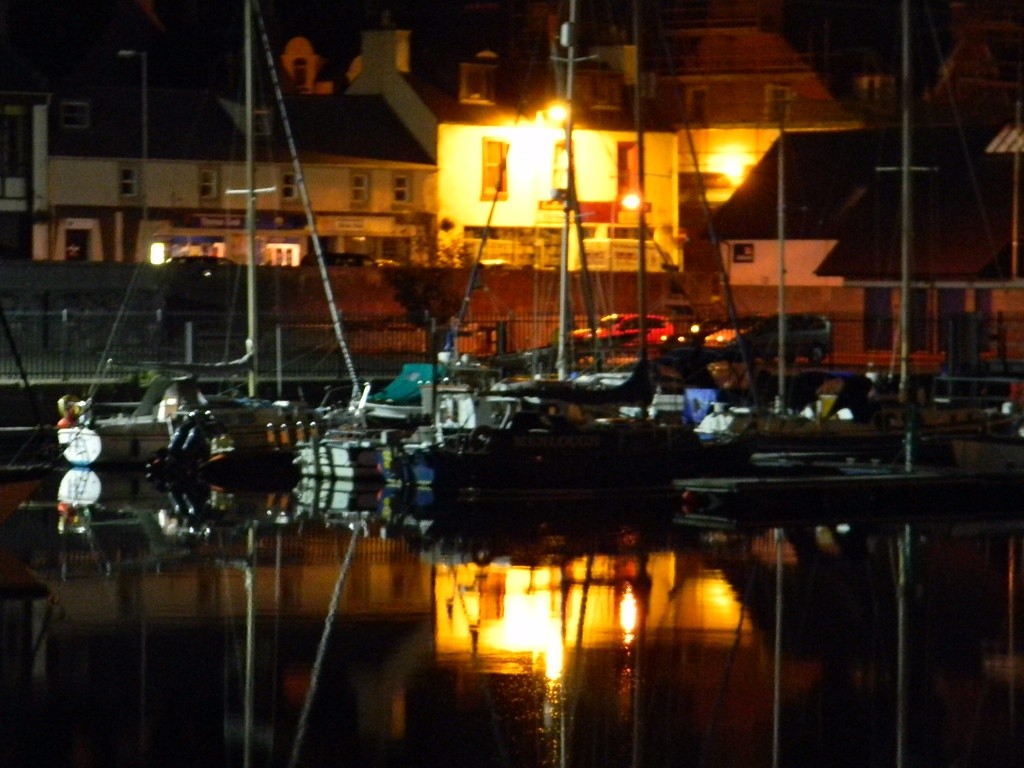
top-left (1, 0), bottom-right (1023, 518)
